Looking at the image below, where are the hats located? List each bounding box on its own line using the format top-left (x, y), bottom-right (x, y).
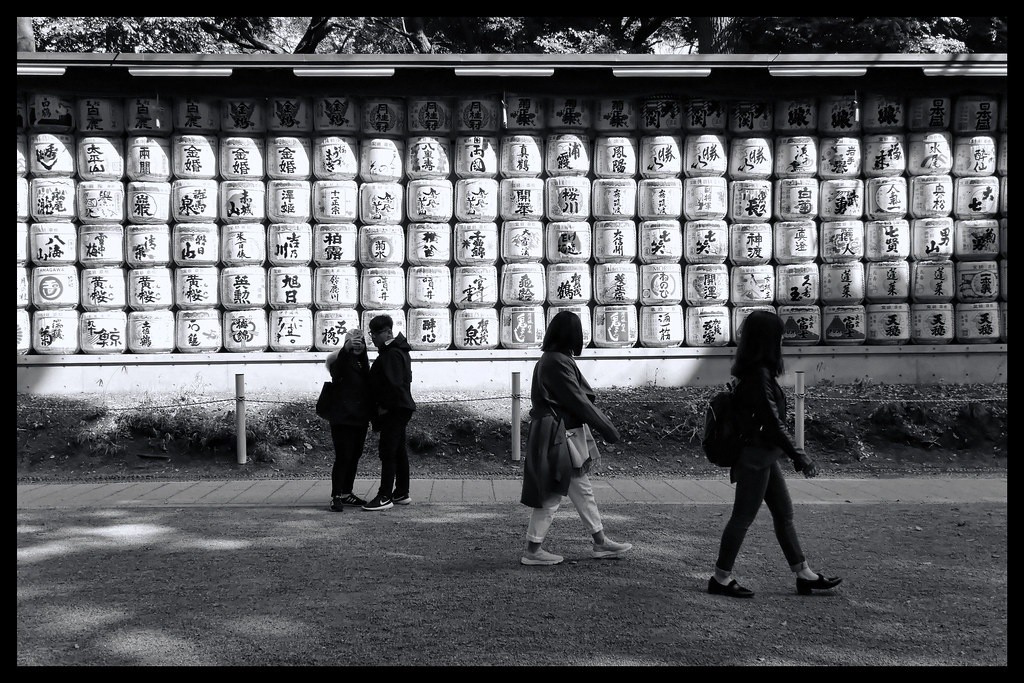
top-left (344, 329), bottom-right (366, 342)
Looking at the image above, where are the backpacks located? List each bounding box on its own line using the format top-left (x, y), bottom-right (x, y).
top-left (702, 376), bottom-right (769, 468)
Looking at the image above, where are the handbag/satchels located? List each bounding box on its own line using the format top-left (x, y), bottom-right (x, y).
top-left (564, 426), bottom-right (601, 469)
top-left (315, 382), bottom-right (367, 426)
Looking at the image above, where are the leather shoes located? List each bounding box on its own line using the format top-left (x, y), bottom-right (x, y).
top-left (707, 575), bottom-right (755, 601)
top-left (796, 573), bottom-right (843, 596)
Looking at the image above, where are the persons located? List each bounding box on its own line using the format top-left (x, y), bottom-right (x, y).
top-left (520, 311), bottom-right (632, 565)
top-left (708, 311), bottom-right (843, 598)
top-left (326, 329), bottom-right (371, 512)
top-left (361, 315), bottom-right (416, 510)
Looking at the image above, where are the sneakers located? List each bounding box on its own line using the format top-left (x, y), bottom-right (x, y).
top-left (591, 536), bottom-right (633, 560)
top-left (328, 493), bottom-right (366, 513)
top-left (520, 548), bottom-right (564, 566)
top-left (361, 490), bottom-right (413, 511)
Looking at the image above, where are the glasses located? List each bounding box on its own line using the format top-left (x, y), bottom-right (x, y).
top-left (368, 328), bottom-right (391, 337)
